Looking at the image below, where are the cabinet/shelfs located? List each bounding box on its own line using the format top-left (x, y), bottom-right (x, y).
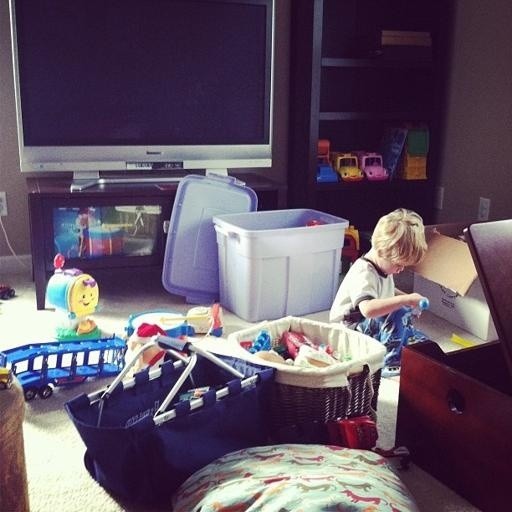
top-left (287, 0), bottom-right (455, 258)
top-left (26, 173), bottom-right (290, 310)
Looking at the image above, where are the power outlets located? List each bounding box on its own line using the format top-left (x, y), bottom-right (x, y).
top-left (0, 191), bottom-right (9, 218)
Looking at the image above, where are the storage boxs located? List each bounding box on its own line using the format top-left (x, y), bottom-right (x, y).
top-left (212, 208), bottom-right (350, 321)
top-left (393, 219), bottom-right (512, 512)
top-left (411, 220), bottom-right (499, 341)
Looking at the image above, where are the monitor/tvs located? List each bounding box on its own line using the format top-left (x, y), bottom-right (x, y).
top-left (9, 0), bottom-right (275, 190)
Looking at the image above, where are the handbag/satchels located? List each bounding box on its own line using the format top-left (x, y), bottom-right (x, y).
top-left (64, 335), bottom-right (277, 512)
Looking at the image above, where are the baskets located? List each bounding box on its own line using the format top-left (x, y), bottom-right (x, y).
top-left (226, 315), bottom-right (388, 448)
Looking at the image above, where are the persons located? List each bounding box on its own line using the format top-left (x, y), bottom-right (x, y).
top-left (328, 208), bottom-right (432, 378)
